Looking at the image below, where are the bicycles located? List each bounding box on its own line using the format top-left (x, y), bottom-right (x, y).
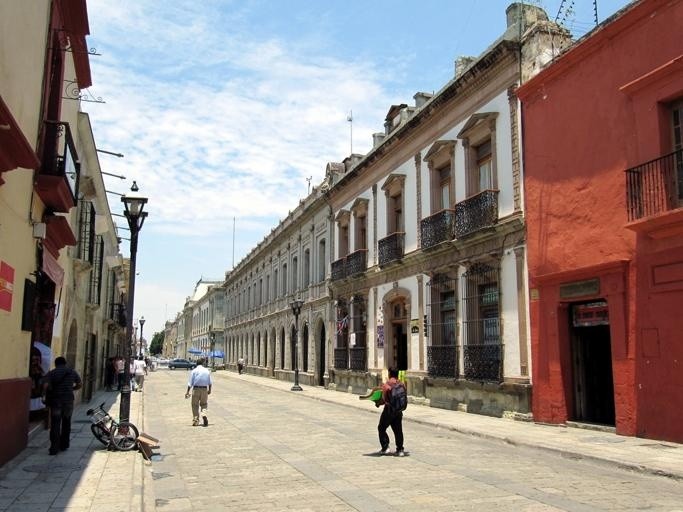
top-left (85, 400), bottom-right (140, 451)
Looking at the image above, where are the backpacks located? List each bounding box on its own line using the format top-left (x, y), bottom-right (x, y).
top-left (386, 380), bottom-right (408, 415)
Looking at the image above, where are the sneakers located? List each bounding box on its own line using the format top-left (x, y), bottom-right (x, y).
top-left (378, 448), bottom-right (391, 456)
top-left (192, 416), bottom-right (199, 427)
top-left (201, 411), bottom-right (208, 427)
top-left (393, 447), bottom-right (405, 457)
top-left (49, 447), bottom-right (67, 455)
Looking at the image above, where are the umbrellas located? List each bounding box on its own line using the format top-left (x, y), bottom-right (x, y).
top-left (188, 347), bottom-right (224, 358)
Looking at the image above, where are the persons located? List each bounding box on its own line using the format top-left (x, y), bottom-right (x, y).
top-left (110, 354), bottom-right (150, 391)
top-left (186, 358), bottom-right (212, 427)
top-left (238, 356), bottom-right (245, 375)
top-left (370, 370), bottom-right (408, 457)
top-left (42, 356), bottom-right (82, 456)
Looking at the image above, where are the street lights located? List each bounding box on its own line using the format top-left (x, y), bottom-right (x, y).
top-left (288, 293), bottom-right (306, 392)
top-left (138, 314), bottom-right (146, 356)
top-left (118, 175), bottom-right (150, 429)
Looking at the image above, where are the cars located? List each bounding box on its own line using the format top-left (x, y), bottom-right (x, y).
top-left (149, 355), bottom-right (225, 371)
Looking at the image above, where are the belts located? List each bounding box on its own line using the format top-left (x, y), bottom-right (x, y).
top-left (193, 385), bottom-right (207, 389)
